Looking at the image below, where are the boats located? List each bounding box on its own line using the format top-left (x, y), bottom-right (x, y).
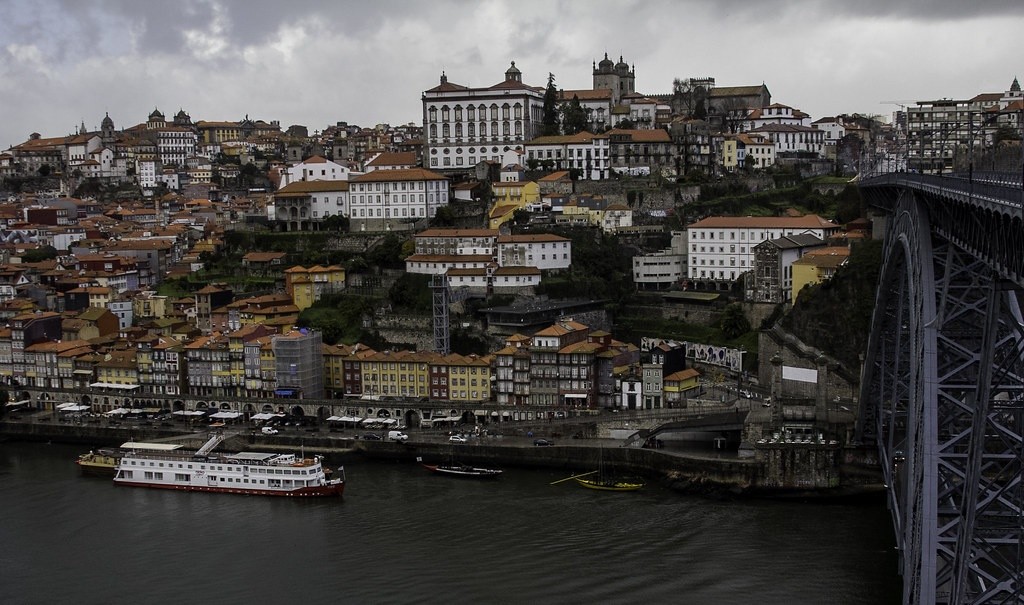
top-left (114, 436), bottom-right (345, 498)
top-left (74, 448), bottom-right (126, 471)
top-left (423, 464), bottom-right (503, 477)
top-left (576, 478), bottom-right (646, 492)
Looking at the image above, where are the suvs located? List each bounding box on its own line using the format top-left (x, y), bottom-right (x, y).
top-left (449, 436), bottom-right (467, 443)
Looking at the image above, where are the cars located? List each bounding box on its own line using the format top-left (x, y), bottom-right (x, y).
top-left (448, 431), bottom-right (464, 438)
top-left (364, 432), bottom-right (380, 440)
top-left (9, 415), bottom-right (23, 421)
top-left (895, 451), bottom-right (906, 462)
top-left (534, 438), bottom-right (555, 446)
top-left (262, 427), bottom-right (279, 435)
top-left (762, 398), bottom-right (771, 407)
top-left (740, 390), bottom-right (753, 398)
top-left (38, 416), bottom-right (51, 424)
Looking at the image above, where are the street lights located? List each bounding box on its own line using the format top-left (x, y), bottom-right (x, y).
top-left (938, 122), bottom-right (971, 177)
top-left (920, 129), bottom-right (947, 174)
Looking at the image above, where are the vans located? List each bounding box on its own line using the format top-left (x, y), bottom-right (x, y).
top-left (389, 431), bottom-right (408, 440)
